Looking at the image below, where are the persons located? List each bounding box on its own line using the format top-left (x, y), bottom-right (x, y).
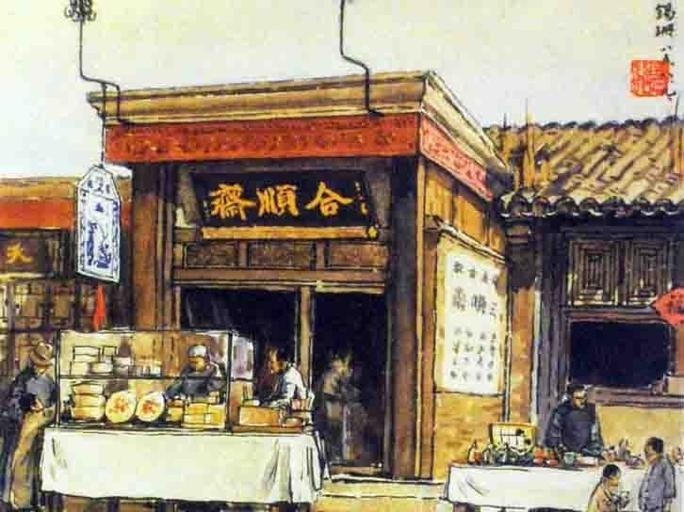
top-left (545, 383), bottom-right (601, 455)
top-left (638, 436), bottom-right (676, 512)
top-left (162, 345), bottom-right (225, 406)
top-left (586, 464), bottom-right (621, 512)
top-left (4, 393), bottom-right (57, 510)
top-left (318, 352), bottom-right (366, 463)
top-left (261, 343), bottom-right (308, 409)
top-left (0, 342), bottom-right (57, 505)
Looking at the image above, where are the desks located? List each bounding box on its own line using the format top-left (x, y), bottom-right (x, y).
top-left (43, 417), bottom-right (315, 512)
top-left (448, 456), bottom-right (680, 512)
